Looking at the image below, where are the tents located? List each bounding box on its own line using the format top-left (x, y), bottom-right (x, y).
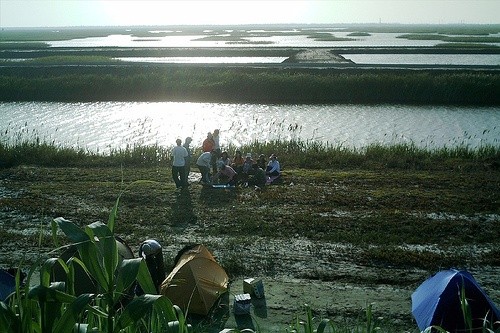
top-left (0, 269), bottom-right (26, 303)
top-left (411, 267), bottom-right (500, 333)
top-left (158, 244), bottom-right (229, 317)
top-left (49, 236), bottom-right (134, 307)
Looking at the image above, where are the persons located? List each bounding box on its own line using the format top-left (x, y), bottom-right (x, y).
top-left (170, 139), bottom-right (188, 192)
top-left (183, 137), bottom-right (192, 186)
top-left (196, 129), bottom-right (281, 190)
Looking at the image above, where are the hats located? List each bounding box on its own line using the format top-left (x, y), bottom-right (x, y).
top-left (269, 154), bottom-right (278, 160)
top-left (217, 161), bottom-right (225, 169)
top-left (246, 153), bottom-right (252, 158)
